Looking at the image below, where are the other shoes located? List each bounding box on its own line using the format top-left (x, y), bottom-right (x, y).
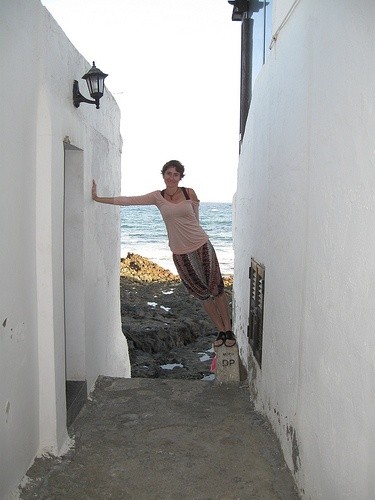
top-left (214, 331), bottom-right (226, 347)
top-left (225, 330), bottom-right (236, 348)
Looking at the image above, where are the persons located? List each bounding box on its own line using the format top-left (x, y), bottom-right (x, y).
top-left (92, 160), bottom-right (238, 347)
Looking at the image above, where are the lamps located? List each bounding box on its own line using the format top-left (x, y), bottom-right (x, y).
top-left (73, 60), bottom-right (108, 109)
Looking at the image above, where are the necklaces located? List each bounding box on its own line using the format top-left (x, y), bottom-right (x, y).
top-left (164, 187), bottom-right (179, 200)
